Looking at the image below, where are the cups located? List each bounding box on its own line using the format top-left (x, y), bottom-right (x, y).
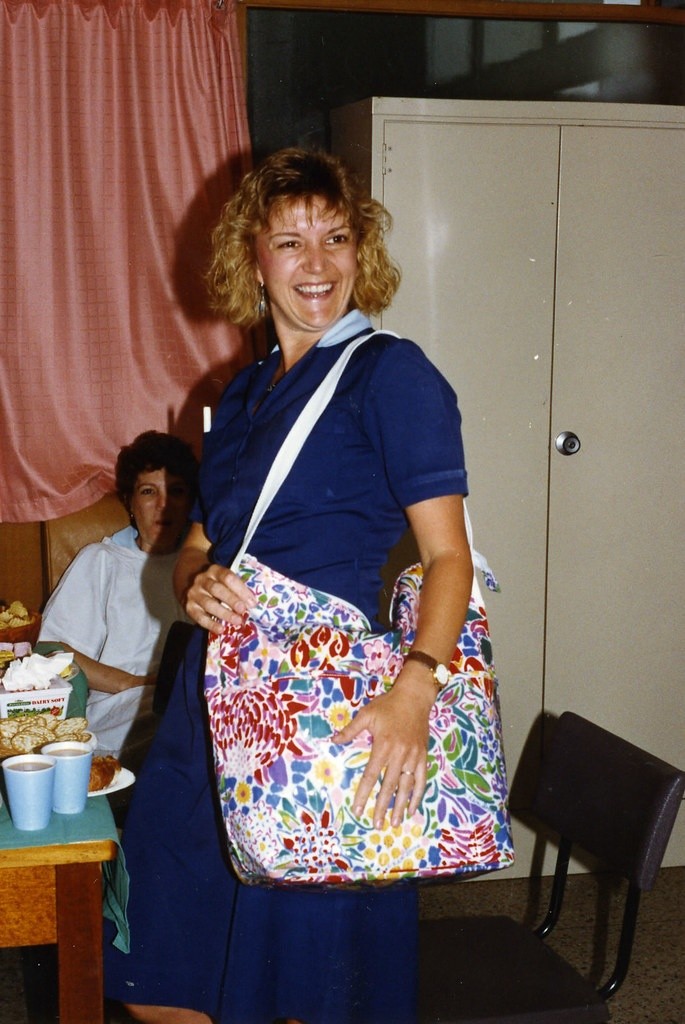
top-left (41, 741), bottom-right (92, 814)
top-left (2, 754), bottom-right (58, 833)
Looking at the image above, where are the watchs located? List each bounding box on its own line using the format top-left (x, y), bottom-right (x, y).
top-left (402, 651), bottom-right (452, 692)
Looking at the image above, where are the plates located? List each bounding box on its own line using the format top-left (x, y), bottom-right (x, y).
top-left (86, 765), bottom-right (136, 797)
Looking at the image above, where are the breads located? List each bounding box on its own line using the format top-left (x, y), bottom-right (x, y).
top-left (86, 753), bottom-right (122, 794)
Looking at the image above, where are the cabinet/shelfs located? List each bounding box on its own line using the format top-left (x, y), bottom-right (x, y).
top-left (327, 93), bottom-right (685, 882)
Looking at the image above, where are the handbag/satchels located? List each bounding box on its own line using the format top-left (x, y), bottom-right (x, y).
top-left (204, 330), bottom-right (515, 888)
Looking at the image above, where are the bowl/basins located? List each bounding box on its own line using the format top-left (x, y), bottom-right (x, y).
top-left (0, 675), bottom-right (74, 720)
top-left (0, 606), bottom-right (43, 651)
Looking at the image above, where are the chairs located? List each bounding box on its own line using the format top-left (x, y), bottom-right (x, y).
top-left (415, 711), bottom-right (684, 1024)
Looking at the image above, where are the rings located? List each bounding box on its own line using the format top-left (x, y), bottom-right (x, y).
top-left (398, 769), bottom-right (418, 778)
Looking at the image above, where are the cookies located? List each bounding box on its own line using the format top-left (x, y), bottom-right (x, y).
top-left (0, 711), bottom-right (90, 760)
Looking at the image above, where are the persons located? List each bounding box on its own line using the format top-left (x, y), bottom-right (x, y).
top-left (33, 429), bottom-right (203, 833)
top-left (113, 147), bottom-right (478, 1023)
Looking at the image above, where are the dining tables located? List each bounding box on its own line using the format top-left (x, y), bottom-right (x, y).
top-left (0, 752), bottom-right (131, 1024)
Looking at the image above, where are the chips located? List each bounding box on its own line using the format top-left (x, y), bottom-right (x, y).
top-left (0, 600), bottom-right (35, 630)
